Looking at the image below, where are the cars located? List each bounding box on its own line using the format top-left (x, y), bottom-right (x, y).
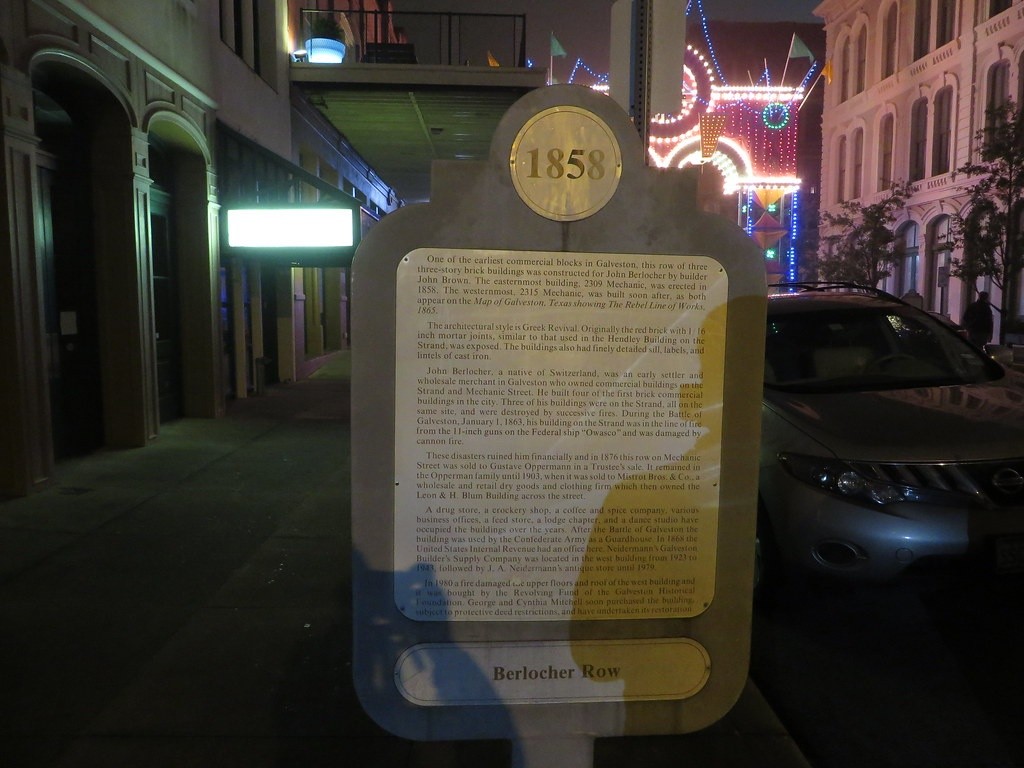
top-left (756, 282), bottom-right (1024, 617)
